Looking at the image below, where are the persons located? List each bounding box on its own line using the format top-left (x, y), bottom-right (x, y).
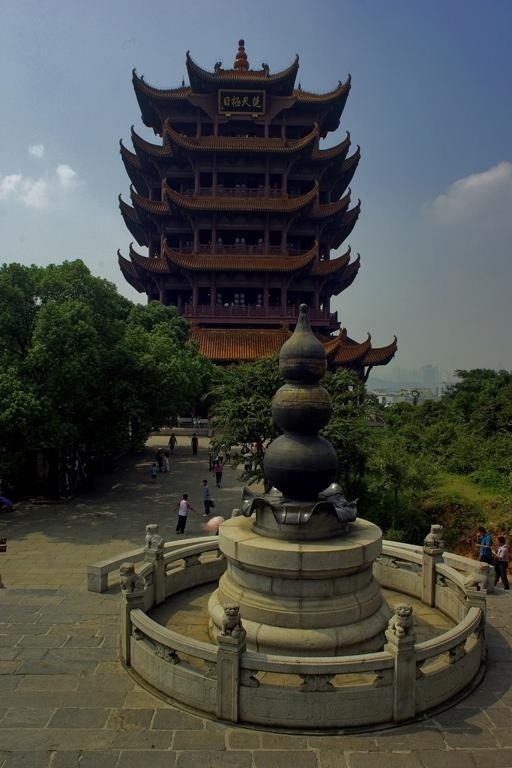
top-left (201, 480), bottom-right (214, 517)
top-left (471, 524), bottom-right (491, 559)
top-left (148, 432), bottom-right (258, 489)
top-left (490, 535), bottom-right (511, 590)
top-left (175, 491), bottom-right (200, 533)
top-left (0, 494), bottom-right (14, 509)
